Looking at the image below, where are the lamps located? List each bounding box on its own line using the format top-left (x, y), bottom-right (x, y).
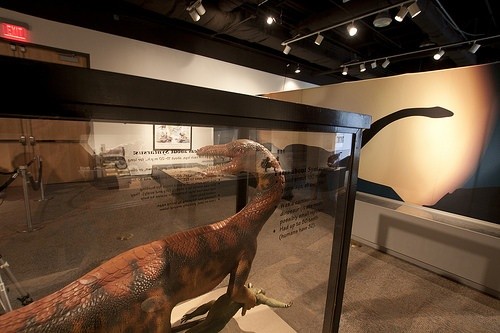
top-left (433, 48), bottom-right (445, 60)
top-left (371, 61), bottom-right (376, 69)
top-left (359, 62), bottom-right (366, 72)
top-left (315, 32), bottom-right (324, 45)
top-left (406, 0), bottom-right (421, 18)
top-left (394, 4), bottom-right (408, 22)
top-left (283, 43), bottom-right (291, 54)
top-left (342, 65), bottom-right (348, 75)
top-left (187, 0), bottom-right (206, 21)
top-left (382, 57), bottom-right (390, 68)
top-left (470, 40), bottom-right (481, 53)
top-left (347, 20), bottom-right (357, 36)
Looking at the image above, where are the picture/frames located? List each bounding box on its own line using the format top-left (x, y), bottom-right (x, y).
top-left (153, 123), bottom-right (192, 150)
